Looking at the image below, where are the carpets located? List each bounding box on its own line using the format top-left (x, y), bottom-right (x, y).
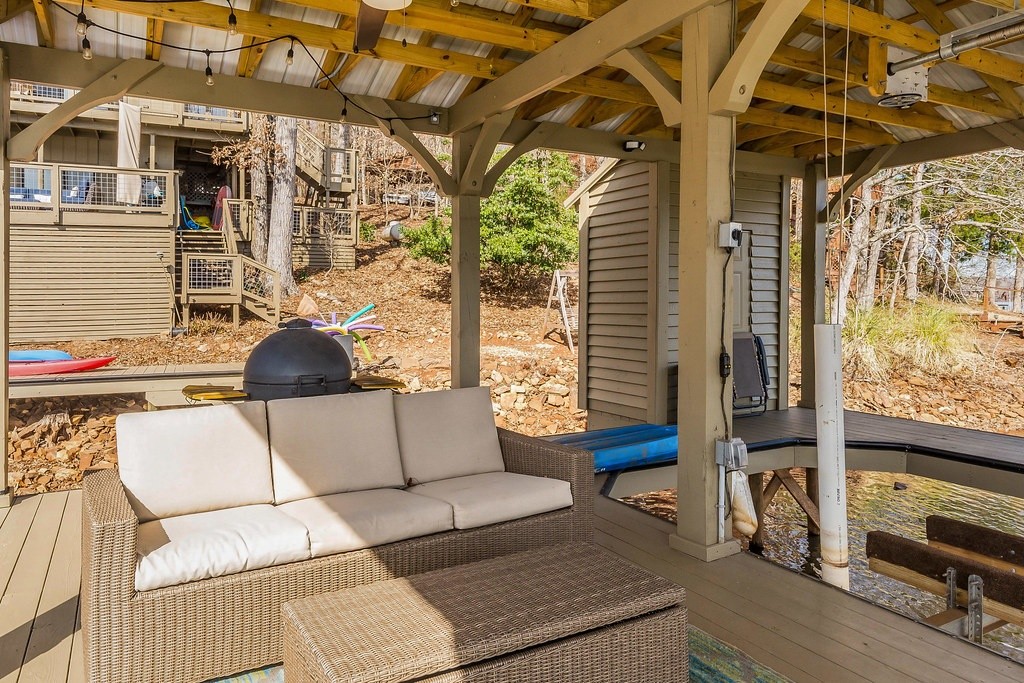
top-left (201, 621), bottom-right (796, 683)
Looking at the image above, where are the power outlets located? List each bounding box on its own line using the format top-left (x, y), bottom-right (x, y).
top-left (718, 222), bottom-right (743, 248)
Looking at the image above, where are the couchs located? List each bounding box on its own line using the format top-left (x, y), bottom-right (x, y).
top-left (10, 180), bottom-right (89, 204)
top-left (81, 387), bottom-right (594, 683)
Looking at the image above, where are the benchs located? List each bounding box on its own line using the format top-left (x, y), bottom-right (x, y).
top-left (282, 539), bottom-right (686, 683)
